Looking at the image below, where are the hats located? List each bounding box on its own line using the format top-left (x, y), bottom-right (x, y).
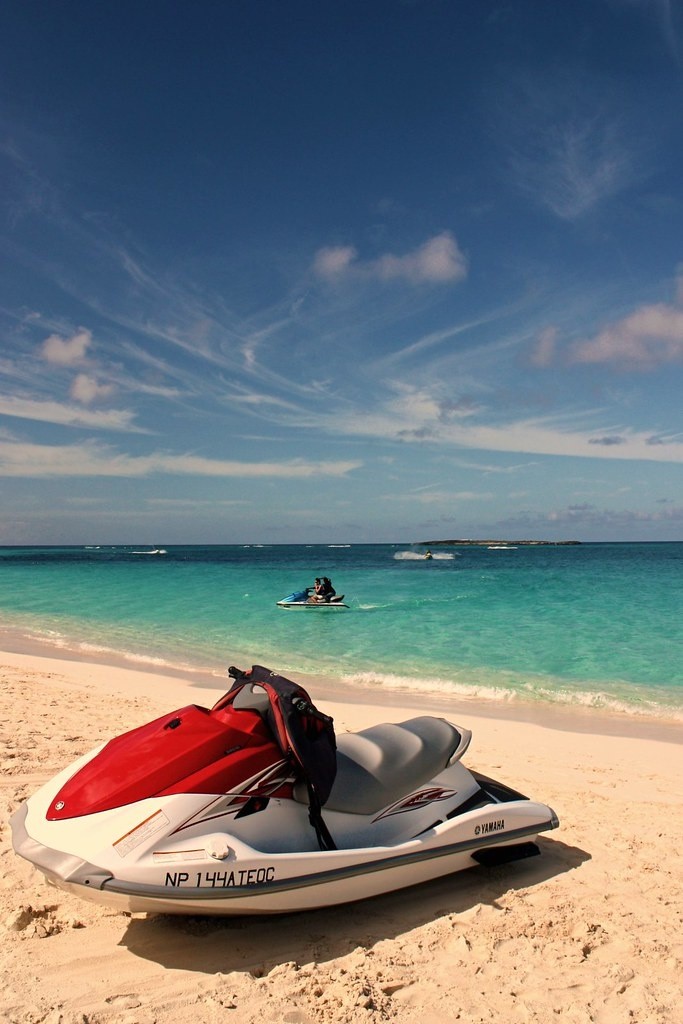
top-left (320, 577), bottom-right (328, 579)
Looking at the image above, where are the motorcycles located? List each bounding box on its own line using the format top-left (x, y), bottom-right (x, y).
top-left (427, 554), bottom-right (434, 560)
top-left (6, 660), bottom-right (558, 918)
top-left (275, 588), bottom-right (351, 612)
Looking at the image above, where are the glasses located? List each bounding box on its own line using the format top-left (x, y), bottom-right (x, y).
top-left (315, 582), bottom-right (318, 583)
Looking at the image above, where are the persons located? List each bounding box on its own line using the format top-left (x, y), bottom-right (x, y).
top-left (323, 576), bottom-right (336, 603)
top-left (307, 577), bottom-right (324, 603)
